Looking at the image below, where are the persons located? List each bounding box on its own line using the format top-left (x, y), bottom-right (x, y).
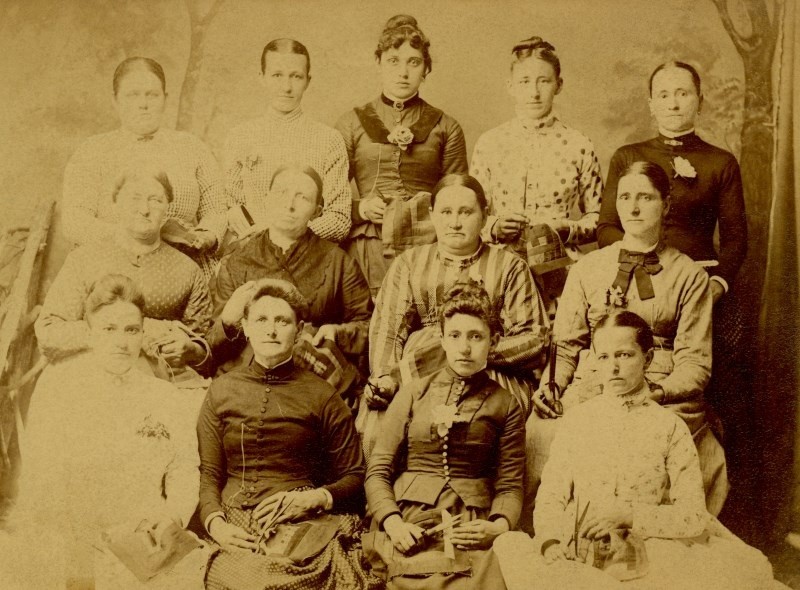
top-left (597, 60), bottom-right (746, 358)
top-left (61, 55), bottom-right (229, 283)
top-left (196, 285), bottom-right (386, 590)
top-left (354, 174), bottom-right (549, 468)
top-left (492, 309), bottom-right (775, 590)
top-left (204, 166), bottom-right (375, 410)
top-left (521, 160), bottom-right (731, 537)
top-left (331, 13), bottom-right (469, 301)
top-left (471, 36), bottom-right (604, 329)
top-left (34, 156), bottom-right (212, 420)
top-left (215, 39), bottom-right (352, 243)
top-left (364, 282), bottom-right (526, 590)
top-left (0, 273), bottom-right (206, 590)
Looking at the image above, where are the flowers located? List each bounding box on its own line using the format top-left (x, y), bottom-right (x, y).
top-left (670, 155), bottom-right (699, 184)
top-left (433, 401), bottom-right (456, 438)
top-left (386, 124), bottom-right (415, 153)
